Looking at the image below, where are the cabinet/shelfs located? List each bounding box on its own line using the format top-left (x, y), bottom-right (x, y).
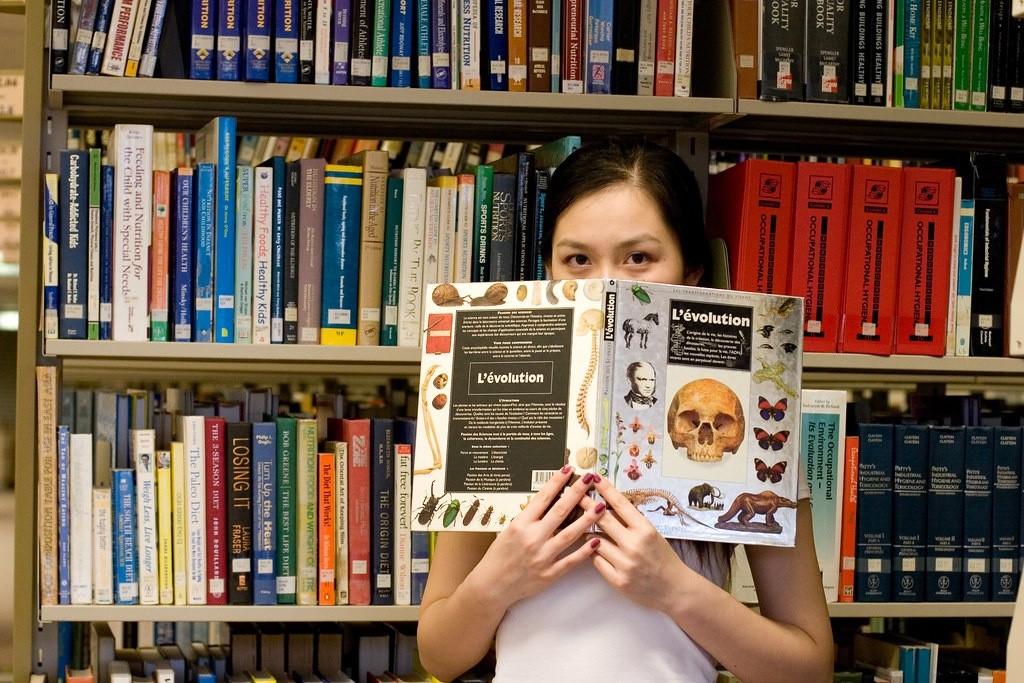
top-left (11, 0), bottom-right (1024, 683)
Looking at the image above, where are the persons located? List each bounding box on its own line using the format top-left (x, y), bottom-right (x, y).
top-left (417, 137), bottom-right (835, 683)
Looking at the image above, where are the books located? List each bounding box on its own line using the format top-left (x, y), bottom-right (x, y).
top-left (721, 382), bottom-right (1024, 602)
top-left (730, 0), bottom-right (1024, 114)
top-left (42, 0), bottom-right (694, 98)
top-left (56, 377), bottom-right (439, 606)
top-left (408, 277), bottom-right (803, 549)
top-left (39, 114), bottom-right (583, 345)
top-left (60, 621), bottom-right (438, 683)
top-left (717, 617), bottom-right (1006, 683)
top-left (707, 145), bottom-right (1024, 359)
